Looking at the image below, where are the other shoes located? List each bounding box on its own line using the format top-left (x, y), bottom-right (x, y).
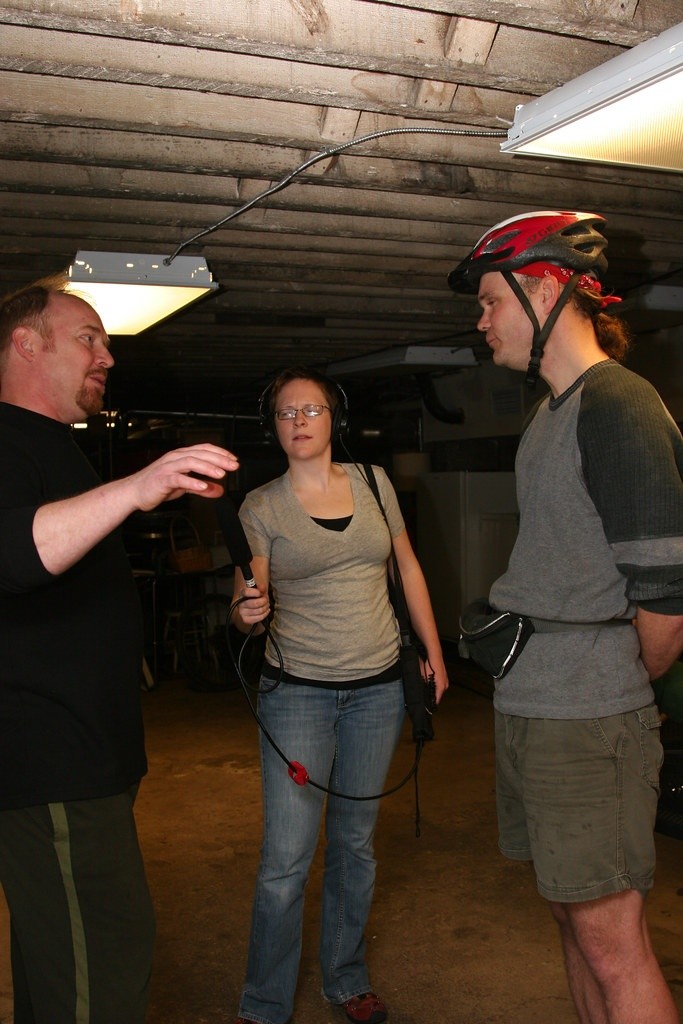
top-left (341, 991), bottom-right (388, 1024)
top-left (234, 1017), bottom-right (259, 1024)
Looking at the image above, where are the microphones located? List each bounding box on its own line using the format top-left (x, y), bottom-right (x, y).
top-left (216, 496), bottom-right (271, 634)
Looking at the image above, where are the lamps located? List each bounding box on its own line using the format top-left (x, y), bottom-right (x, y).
top-left (497, 21), bottom-right (683, 173)
top-left (326, 345), bottom-right (480, 378)
top-left (69, 251), bottom-right (220, 336)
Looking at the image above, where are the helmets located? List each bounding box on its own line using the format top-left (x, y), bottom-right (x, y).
top-left (447, 210), bottom-right (609, 292)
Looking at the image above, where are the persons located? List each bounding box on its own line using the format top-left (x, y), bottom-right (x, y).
top-left (447, 211), bottom-right (683, 1024)
top-left (232, 363), bottom-right (449, 1024)
top-left (0, 287), bottom-right (240, 1024)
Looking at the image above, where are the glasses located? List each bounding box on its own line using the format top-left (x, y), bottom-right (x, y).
top-left (274, 404), bottom-right (333, 420)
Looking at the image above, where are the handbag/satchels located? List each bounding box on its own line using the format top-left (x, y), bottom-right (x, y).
top-left (457, 595), bottom-right (633, 680)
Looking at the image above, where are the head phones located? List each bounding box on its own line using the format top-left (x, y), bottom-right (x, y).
top-left (259, 374), bottom-right (350, 443)
